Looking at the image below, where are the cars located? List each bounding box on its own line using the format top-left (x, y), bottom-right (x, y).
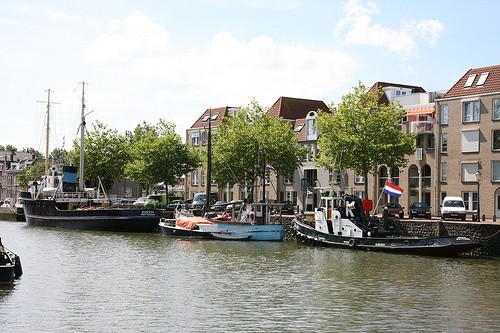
top-left (381, 202), bottom-right (405, 219)
top-left (114, 194), bottom-right (296, 216)
top-left (409, 202), bottom-right (432, 219)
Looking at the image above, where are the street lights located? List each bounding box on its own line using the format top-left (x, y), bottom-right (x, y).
top-left (475, 170), bottom-right (481, 222)
top-left (202, 107), bottom-right (219, 208)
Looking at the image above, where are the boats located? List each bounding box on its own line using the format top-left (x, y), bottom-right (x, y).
top-left (161, 203), bottom-right (288, 243)
top-left (0, 237), bottom-right (23, 287)
top-left (290, 192), bottom-right (480, 259)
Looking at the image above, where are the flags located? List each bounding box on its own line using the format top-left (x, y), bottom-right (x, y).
top-left (383, 181), bottom-right (404, 198)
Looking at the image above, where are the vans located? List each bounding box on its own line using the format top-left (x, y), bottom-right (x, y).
top-left (440, 196), bottom-right (467, 221)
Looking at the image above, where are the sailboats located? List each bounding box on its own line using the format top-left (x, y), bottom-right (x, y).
top-left (22, 81), bottom-right (168, 232)
